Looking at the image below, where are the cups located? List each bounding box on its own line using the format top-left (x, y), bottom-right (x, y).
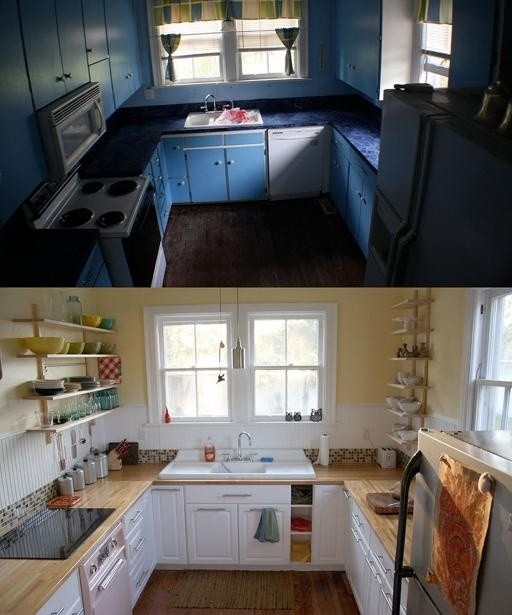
top-left (38, 410), bottom-right (55, 429)
top-left (51, 390), bottom-right (101, 425)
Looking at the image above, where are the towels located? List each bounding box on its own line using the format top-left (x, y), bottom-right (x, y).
top-left (265, 507), bottom-right (280, 542)
top-left (254, 510), bottom-right (266, 543)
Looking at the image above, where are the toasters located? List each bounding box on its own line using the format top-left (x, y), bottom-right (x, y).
top-left (376, 447), bottom-right (397, 469)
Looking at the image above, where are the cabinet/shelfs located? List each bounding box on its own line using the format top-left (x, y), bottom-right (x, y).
top-left (267, 126), bottom-right (326, 201)
top-left (123, 488), bottom-right (159, 609)
top-left (183, 130), bottom-right (269, 204)
top-left (152, 486), bottom-right (187, 563)
top-left (32, 570), bottom-right (84, 614)
top-left (342, 488), bottom-right (407, 615)
top-left (289, 483), bottom-right (291, 530)
top-left (330, 128), bottom-right (380, 260)
top-left (184, 483), bottom-right (291, 564)
top-left (11, 303), bottom-right (124, 432)
top-left (143, 138), bottom-right (172, 239)
top-left (382, 289), bottom-right (435, 446)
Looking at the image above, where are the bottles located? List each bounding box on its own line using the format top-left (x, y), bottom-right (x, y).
top-left (66, 467), bottom-right (86, 490)
top-left (55, 391), bottom-right (97, 423)
top-left (204, 435), bottom-right (216, 461)
top-left (58, 475), bottom-right (74, 496)
top-left (402, 343), bottom-right (409, 356)
top-left (67, 294), bottom-right (83, 325)
top-left (74, 458), bottom-right (97, 484)
top-left (97, 387), bottom-right (119, 410)
top-left (419, 342), bottom-right (428, 356)
top-left (398, 348), bottom-right (404, 358)
top-left (85, 451), bottom-right (109, 478)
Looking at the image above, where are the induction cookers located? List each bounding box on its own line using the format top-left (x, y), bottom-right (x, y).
top-left (2, 506), bottom-right (117, 561)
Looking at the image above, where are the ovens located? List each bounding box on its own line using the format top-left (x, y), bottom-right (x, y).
top-left (79, 522), bottom-right (134, 615)
top-left (35, 82), bottom-right (107, 176)
top-left (122, 176), bottom-right (164, 289)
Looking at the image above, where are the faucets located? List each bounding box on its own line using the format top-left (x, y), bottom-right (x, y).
top-left (221, 432), bottom-right (257, 463)
top-left (200, 94), bottom-right (217, 112)
top-left (223, 98), bottom-right (234, 110)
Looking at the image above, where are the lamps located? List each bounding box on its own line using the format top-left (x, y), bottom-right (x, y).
top-left (231, 288), bottom-right (245, 368)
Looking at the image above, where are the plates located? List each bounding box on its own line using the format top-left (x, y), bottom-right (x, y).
top-left (28, 378), bottom-right (66, 396)
top-left (66, 382), bottom-right (81, 392)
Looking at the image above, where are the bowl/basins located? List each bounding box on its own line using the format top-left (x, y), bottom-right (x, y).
top-left (398, 430), bottom-right (419, 442)
top-left (392, 423), bottom-right (410, 439)
top-left (396, 371), bottom-right (411, 384)
top-left (99, 379), bottom-right (116, 387)
top-left (100, 318), bottom-right (116, 329)
top-left (25, 335), bottom-right (67, 354)
top-left (69, 340), bottom-right (83, 355)
top-left (103, 341), bottom-right (117, 355)
top-left (57, 342), bottom-right (71, 355)
top-left (385, 395), bottom-right (407, 412)
top-left (403, 376), bottom-right (422, 386)
top-left (398, 398), bottom-right (422, 414)
top-left (83, 314), bottom-right (105, 327)
top-left (79, 382), bottom-right (98, 391)
top-left (70, 376), bottom-right (97, 383)
top-left (85, 340), bottom-right (102, 354)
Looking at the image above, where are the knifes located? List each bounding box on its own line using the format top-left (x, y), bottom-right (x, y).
top-left (115, 438), bottom-right (130, 459)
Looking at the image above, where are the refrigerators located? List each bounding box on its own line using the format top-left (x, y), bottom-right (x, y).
top-left (393, 429), bottom-right (511, 611)
top-left (363, 89), bottom-right (512, 287)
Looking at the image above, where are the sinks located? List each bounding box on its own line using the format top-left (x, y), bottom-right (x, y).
top-left (159, 449), bottom-right (316, 480)
top-left (184, 108), bottom-right (263, 128)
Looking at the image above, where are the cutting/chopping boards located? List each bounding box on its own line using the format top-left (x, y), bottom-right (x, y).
top-left (365, 491), bottom-right (415, 515)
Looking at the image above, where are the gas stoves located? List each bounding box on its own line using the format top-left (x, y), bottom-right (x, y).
top-left (21, 173), bottom-right (153, 238)
